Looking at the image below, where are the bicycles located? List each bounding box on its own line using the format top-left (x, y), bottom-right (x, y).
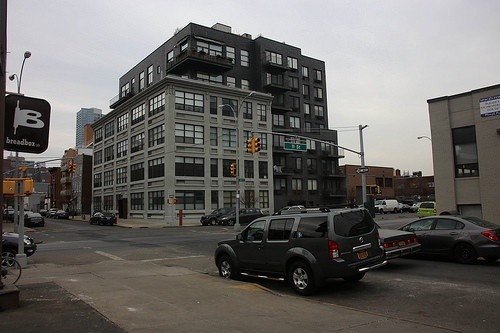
top-left (0, 256), bottom-right (22, 287)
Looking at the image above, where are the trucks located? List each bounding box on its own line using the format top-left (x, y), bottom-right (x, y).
top-left (271, 205), bottom-right (420, 261)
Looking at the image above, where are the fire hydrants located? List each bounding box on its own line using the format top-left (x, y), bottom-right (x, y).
top-left (176, 209), bottom-right (185, 226)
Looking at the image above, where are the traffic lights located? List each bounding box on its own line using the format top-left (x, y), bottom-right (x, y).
top-left (254, 137), bottom-right (261, 154)
top-left (230, 163), bottom-right (234, 175)
top-left (246, 137), bottom-right (254, 155)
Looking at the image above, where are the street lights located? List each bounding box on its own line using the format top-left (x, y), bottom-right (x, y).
top-left (217, 90), bottom-right (257, 233)
top-left (9, 51), bottom-right (32, 271)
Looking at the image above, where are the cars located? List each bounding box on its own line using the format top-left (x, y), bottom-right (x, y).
top-left (396, 215), bottom-right (500, 263)
top-left (0, 231), bottom-right (37, 257)
top-left (2, 208), bottom-right (45, 228)
top-left (375, 199), bottom-right (399, 213)
top-left (200, 208), bottom-right (265, 226)
top-left (214, 209), bottom-right (388, 296)
top-left (88, 211), bottom-right (115, 226)
top-left (417, 201), bottom-right (436, 218)
top-left (41, 209), bottom-right (69, 220)
